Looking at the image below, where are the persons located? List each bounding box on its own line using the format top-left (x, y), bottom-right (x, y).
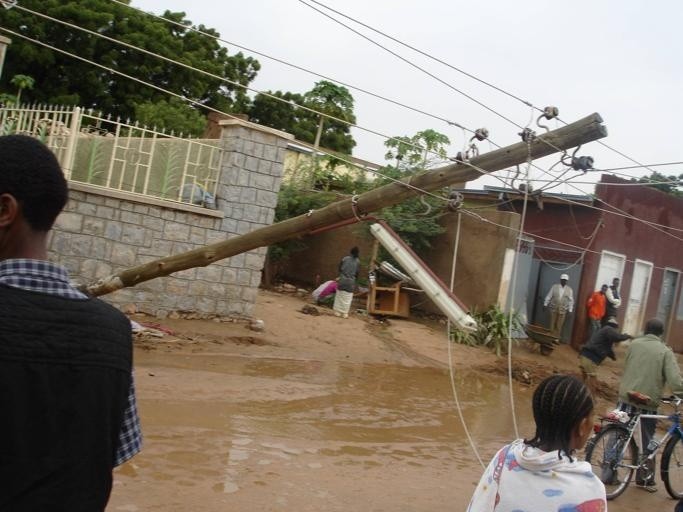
top-left (311, 277), bottom-right (340, 309)
top-left (332, 247), bottom-right (361, 318)
top-left (604, 277), bottom-right (622, 326)
top-left (600, 317), bottom-right (683, 487)
top-left (543, 273), bottom-right (574, 346)
top-left (0, 134), bottom-right (142, 511)
top-left (465, 374), bottom-right (607, 512)
top-left (587, 285), bottom-right (609, 333)
top-left (577, 318), bottom-right (635, 405)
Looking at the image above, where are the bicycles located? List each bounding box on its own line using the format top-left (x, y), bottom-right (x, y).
top-left (585, 391), bottom-right (683, 500)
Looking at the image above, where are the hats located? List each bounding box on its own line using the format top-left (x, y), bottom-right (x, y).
top-left (559, 273), bottom-right (569, 281)
top-left (607, 318), bottom-right (618, 325)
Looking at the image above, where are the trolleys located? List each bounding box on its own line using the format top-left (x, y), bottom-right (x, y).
top-left (514, 315), bottom-right (559, 355)
